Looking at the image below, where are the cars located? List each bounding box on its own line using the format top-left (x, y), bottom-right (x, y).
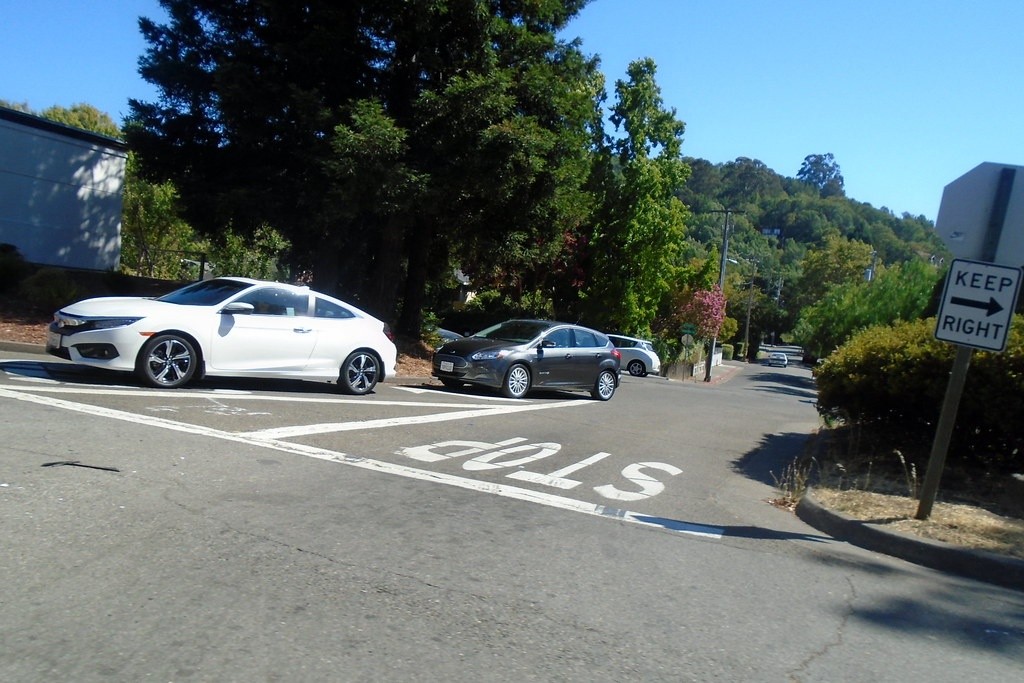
top-left (607, 332), bottom-right (662, 377)
top-left (45, 275), bottom-right (397, 395)
top-left (429, 318), bottom-right (622, 401)
top-left (767, 352), bottom-right (788, 368)
top-left (430, 323), bottom-right (464, 353)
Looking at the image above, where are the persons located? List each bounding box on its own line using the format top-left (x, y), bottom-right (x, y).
top-left (258, 297), bottom-right (271, 313)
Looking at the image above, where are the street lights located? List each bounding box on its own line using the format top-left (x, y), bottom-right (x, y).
top-left (705, 208), bottom-right (746, 382)
